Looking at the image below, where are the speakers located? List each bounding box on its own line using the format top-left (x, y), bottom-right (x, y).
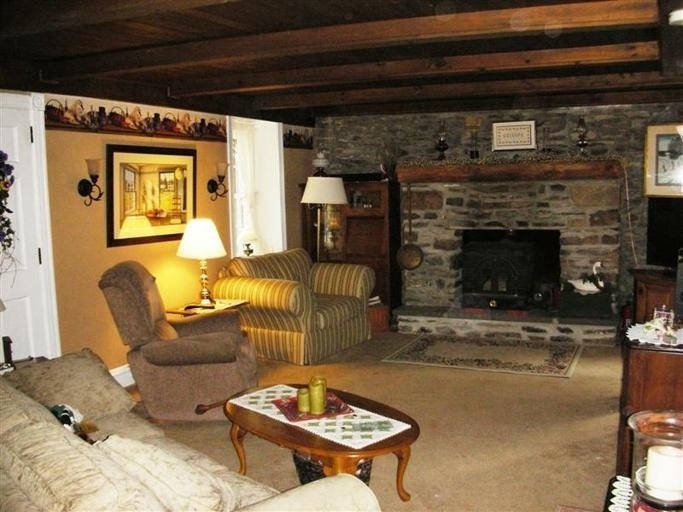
top-left (646, 196), bottom-right (683, 267)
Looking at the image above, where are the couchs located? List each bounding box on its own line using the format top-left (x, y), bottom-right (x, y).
top-left (2, 349), bottom-right (382, 510)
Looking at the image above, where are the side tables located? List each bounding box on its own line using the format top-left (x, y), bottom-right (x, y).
top-left (161, 294), bottom-right (251, 342)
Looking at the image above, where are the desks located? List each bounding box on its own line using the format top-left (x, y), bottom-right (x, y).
top-left (222, 384), bottom-right (419, 503)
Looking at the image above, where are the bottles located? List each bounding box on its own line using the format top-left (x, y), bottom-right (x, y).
top-left (362, 199), bottom-right (375, 208)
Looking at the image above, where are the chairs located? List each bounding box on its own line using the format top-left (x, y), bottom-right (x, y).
top-left (213, 247), bottom-right (376, 366)
top-left (98, 259), bottom-right (258, 420)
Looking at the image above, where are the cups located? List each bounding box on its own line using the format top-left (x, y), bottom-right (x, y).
top-left (627, 410), bottom-right (683, 508)
top-left (297, 376), bottom-right (327, 415)
top-left (653, 305), bottom-right (674, 330)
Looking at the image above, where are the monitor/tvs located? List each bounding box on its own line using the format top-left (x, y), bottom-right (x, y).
top-left (460, 227), bottom-right (561, 311)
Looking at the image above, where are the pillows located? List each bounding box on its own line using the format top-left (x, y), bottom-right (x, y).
top-left (93, 436), bottom-right (242, 512)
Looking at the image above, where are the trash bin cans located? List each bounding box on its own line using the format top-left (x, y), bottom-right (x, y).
top-left (293, 453), bottom-right (371, 486)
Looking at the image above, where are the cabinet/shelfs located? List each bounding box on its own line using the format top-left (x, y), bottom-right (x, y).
top-left (298, 174), bottom-right (401, 330)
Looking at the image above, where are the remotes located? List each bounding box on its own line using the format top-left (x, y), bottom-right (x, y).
top-left (185, 303), bottom-right (215, 311)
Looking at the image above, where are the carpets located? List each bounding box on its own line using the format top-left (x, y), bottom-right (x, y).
top-left (381, 335), bottom-right (582, 379)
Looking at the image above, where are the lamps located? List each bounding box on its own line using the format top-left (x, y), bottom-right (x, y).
top-left (300, 177), bottom-right (348, 263)
top-left (177, 216), bottom-right (228, 304)
top-left (208, 162), bottom-right (227, 201)
top-left (77, 159), bottom-right (103, 207)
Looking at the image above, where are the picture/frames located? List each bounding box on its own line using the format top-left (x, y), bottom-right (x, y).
top-left (491, 119), bottom-right (537, 150)
top-left (641, 122), bottom-right (683, 200)
top-left (104, 144), bottom-right (198, 250)
